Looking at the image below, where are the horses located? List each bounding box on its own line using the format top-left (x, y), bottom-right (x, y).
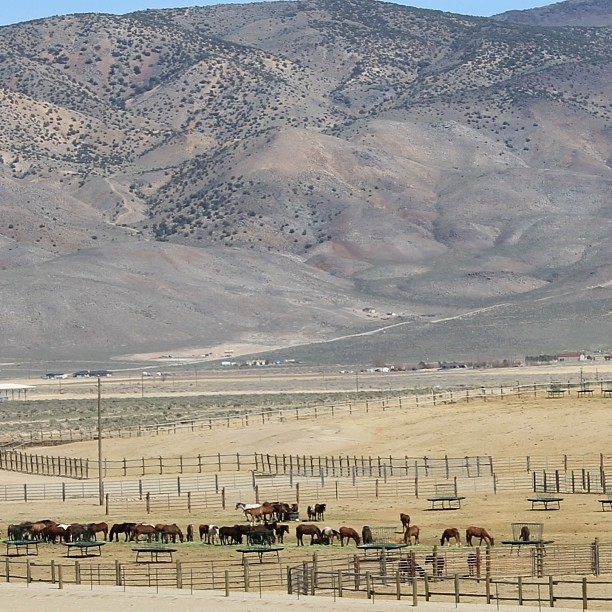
top-left (295, 524), bottom-right (322, 547)
top-left (7, 519), bottom-right (108, 544)
top-left (209, 525), bottom-right (221, 546)
top-left (161, 524), bottom-right (184, 544)
top-left (186, 524), bottom-right (196, 542)
top-left (467, 553), bottom-right (483, 577)
top-left (155, 523), bottom-right (168, 541)
top-left (397, 558), bottom-right (431, 583)
top-left (109, 523), bottom-right (133, 542)
top-left (424, 554), bottom-right (447, 581)
top-left (199, 524), bottom-right (210, 543)
top-left (219, 522), bottom-right (278, 547)
top-left (135, 525), bottom-right (159, 543)
top-left (123, 522), bottom-right (142, 541)
top-left (465, 526), bottom-right (495, 549)
top-left (235, 500), bottom-right (299, 526)
top-left (439, 528), bottom-right (463, 547)
top-left (362, 526), bottom-right (374, 544)
top-left (399, 513), bottom-right (411, 534)
top-left (315, 503), bottom-right (326, 522)
top-left (339, 527), bottom-right (361, 548)
top-left (307, 505), bottom-right (317, 521)
top-left (321, 527), bottom-right (341, 545)
top-left (276, 524), bottom-right (290, 544)
top-left (518, 526), bottom-right (531, 542)
top-left (403, 525), bottom-right (420, 546)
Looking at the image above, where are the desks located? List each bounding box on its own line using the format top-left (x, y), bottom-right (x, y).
top-left (62, 541), bottom-right (106, 556)
top-left (546, 390), bottom-right (565, 397)
top-left (502, 540), bottom-right (556, 554)
top-left (602, 389), bottom-right (612, 397)
top-left (427, 496), bottom-right (466, 508)
top-left (3, 539), bottom-right (40, 555)
top-left (235, 546), bottom-right (285, 562)
top-left (131, 547), bottom-right (177, 562)
top-left (598, 498), bottom-right (612, 511)
top-left (576, 389), bottom-right (595, 397)
top-left (526, 497), bottom-right (563, 510)
top-left (358, 542), bottom-right (408, 558)
